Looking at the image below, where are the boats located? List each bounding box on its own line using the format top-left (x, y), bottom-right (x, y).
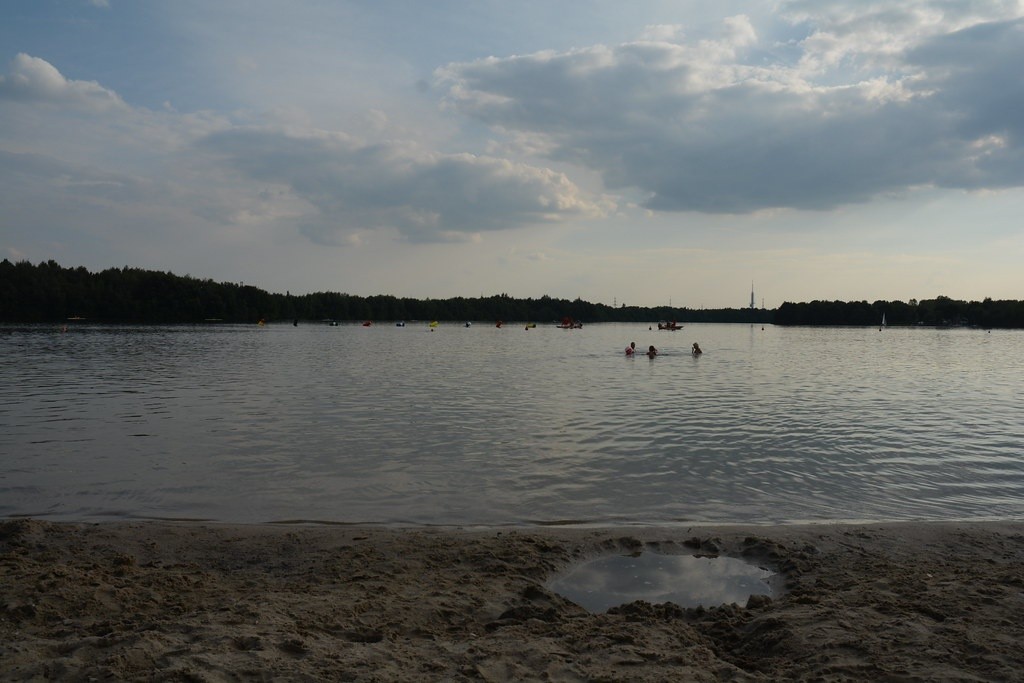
top-left (556, 319), bottom-right (583, 329)
top-left (658, 320), bottom-right (684, 330)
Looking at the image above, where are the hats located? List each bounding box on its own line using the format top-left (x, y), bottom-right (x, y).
top-left (693, 343), bottom-right (698, 346)
top-left (625, 346), bottom-right (631, 352)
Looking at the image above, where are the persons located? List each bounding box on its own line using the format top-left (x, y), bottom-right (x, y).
top-left (625, 342), bottom-right (636, 353)
top-left (692, 343), bottom-right (702, 353)
top-left (646, 346), bottom-right (656, 354)
